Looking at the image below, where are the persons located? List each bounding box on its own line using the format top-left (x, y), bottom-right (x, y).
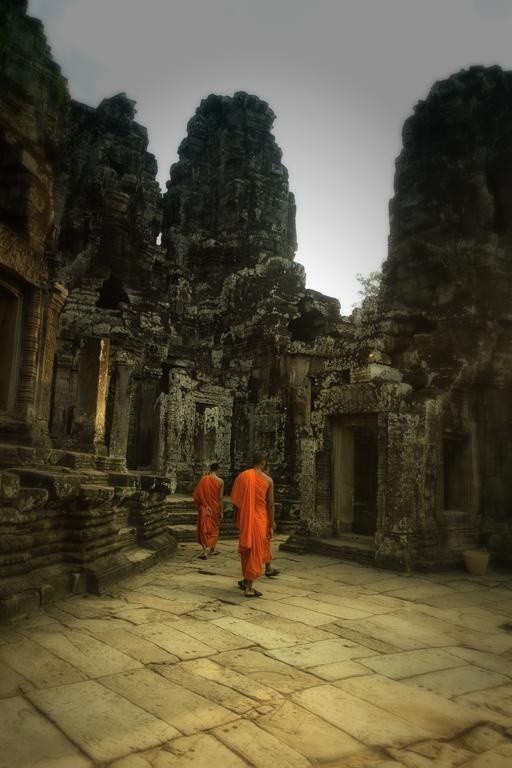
top-left (265, 557), bottom-right (280, 577)
top-left (229, 451), bottom-right (276, 597)
top-left (193, 463), bottom-right (225, 561)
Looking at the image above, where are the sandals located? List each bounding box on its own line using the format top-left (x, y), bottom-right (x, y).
top-left (209, 551), bottom-right (220, 555)
top-left (265, 569), bottom-right (279, 576)
top-left (198, 556), bottom-right (208, 560)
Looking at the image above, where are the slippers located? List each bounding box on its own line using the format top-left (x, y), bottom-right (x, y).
top-left (238, 581), bottom-right (262, 597)
